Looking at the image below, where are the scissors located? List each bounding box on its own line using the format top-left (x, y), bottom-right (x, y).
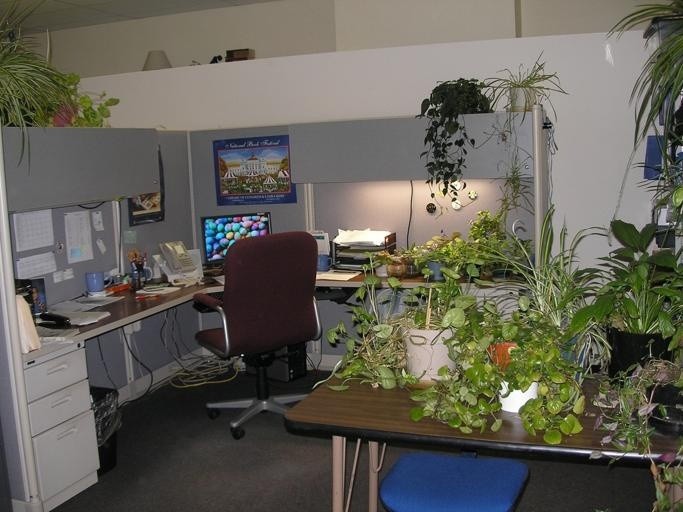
top-left (135, 294), bottom-right (159, 299)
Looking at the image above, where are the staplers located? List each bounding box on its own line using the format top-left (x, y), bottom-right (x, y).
top-left (38, 312), bottom-right (71, 329)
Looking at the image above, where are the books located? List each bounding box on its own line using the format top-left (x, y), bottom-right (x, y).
top-left (15, 294), bottom-right (42, 354)
top-left (134, 286), bottom-right (182, 295)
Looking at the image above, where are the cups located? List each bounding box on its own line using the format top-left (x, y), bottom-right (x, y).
top-left (85, 271), bottom-right (113, 292)
top-left (315, 254), bottom-right (332, 272)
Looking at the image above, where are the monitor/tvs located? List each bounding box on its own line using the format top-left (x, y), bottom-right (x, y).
top-left (200, 211), bottom-right (273, 265)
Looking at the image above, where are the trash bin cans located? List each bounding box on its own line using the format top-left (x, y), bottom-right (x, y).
top-left (89, 385), bottom-right (119, 476)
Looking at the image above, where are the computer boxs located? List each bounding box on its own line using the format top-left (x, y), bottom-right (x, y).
top-left (246, 342), bottom-right (306, 384)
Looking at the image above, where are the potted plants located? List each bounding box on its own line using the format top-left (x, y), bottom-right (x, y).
top-left (376, 209), bottom-right (533, 283)
top-left (309, 205), bottom-right (683, 512)
top-left (414, 47), bottom-right (571, 229)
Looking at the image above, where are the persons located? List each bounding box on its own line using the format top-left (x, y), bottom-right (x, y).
top-left (30, 286), bottom-right (47, 315)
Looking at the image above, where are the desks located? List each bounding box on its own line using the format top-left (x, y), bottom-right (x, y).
top-left (282, 373), bottom-right (683, 511)
top-left (48, 267), bottom-right (474, 341)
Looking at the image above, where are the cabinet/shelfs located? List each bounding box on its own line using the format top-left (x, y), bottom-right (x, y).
top-left (24, 347), bottom-right (103, 511)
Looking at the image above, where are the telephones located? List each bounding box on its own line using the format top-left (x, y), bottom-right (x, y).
top-left (158, 240), bottom-right (196, 273)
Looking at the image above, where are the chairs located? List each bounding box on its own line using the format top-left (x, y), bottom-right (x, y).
top-left (193, 232), bottom-right (323, 440)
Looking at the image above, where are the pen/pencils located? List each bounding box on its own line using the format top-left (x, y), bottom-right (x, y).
top-left (131, 252), bottom-right (147, 272)
